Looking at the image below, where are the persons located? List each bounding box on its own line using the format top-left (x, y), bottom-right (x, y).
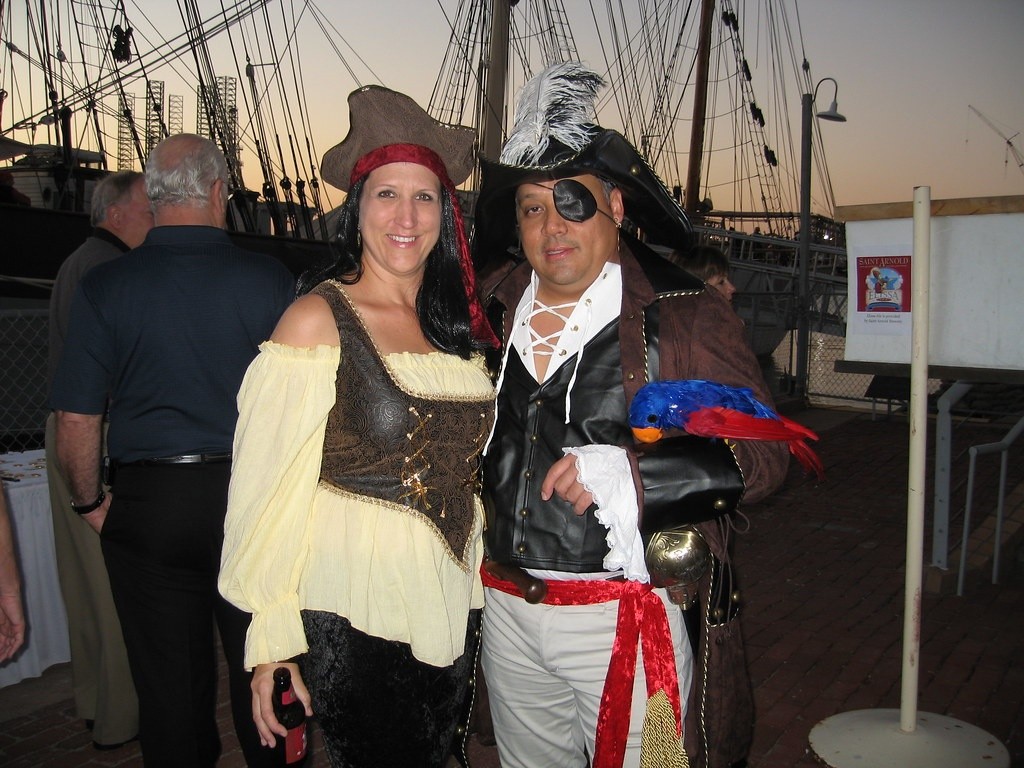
top-left (44, 164), bottom-right (159, 750)
top-left (217, 85), bottom-right (498, 768)
top-left (472, 63), bottom-right (791, 765)
top-left (50, 129), bottom-right (324, 768)
top-left (667, 244), bottom-right (737, 307)
top-left (0, 482), bottom-right (30, 667)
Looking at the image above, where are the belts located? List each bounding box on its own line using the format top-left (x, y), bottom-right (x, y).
top-left (144, 451), bottom-right (232, 467)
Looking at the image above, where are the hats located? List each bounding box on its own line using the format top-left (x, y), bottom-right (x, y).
top-left (318, 83), bottom-right (478, 194)
top-left (470, 60), bottom-right (693, 255)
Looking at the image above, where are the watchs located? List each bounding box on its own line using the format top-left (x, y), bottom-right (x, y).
top-left (69, 492), bottom-right (105, 515)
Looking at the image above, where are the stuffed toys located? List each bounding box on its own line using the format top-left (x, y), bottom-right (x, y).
top-left (628, 376), bottom-right (828, 479)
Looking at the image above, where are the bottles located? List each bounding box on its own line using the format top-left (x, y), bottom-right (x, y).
top-left (270, 668), bottom-right (309, 767)
